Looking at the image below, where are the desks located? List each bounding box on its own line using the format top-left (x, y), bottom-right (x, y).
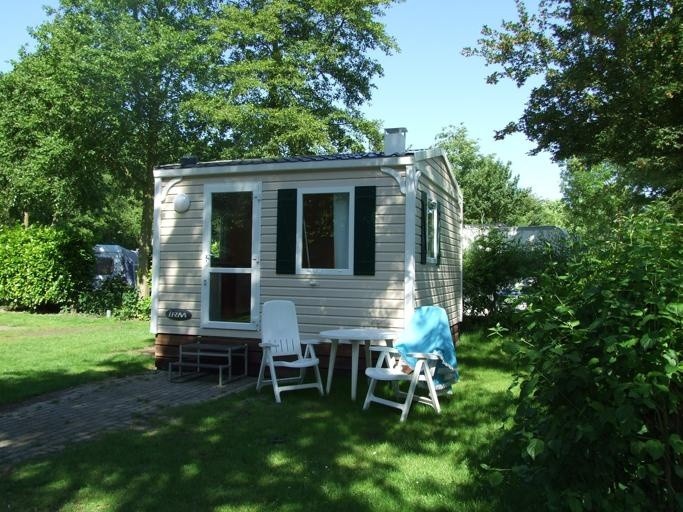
top-left (320, 327), bottom-right (401, 401)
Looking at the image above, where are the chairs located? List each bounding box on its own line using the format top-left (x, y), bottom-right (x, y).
top-left (361, 305), bottom-right (456, 422)
top-left (256, 299), bottom-right (324, 405)
